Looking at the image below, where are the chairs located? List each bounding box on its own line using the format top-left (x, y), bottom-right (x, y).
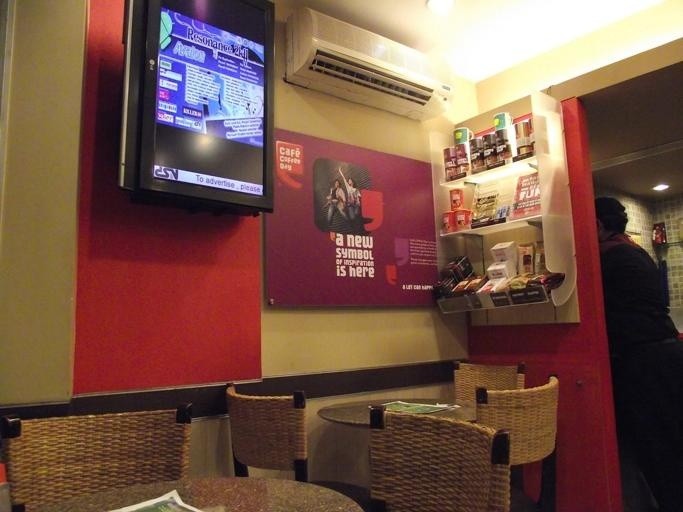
top-left (226, 382), bottom-right (308, 483)
top-left (475, 373), bottom-right (563, 511)
top-left (5, 402), bottom-right (191, 504)
top-left (451, 359), bottom-right (525, 404)
top-left (366, 403), bottom-right (512, 512)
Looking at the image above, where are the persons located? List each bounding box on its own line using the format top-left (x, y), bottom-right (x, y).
top-left (594, 196), bottom-right (682, 511)
top-left (325, 178), bottom-right (348, 226)
top-left (337, 166), bottom-right (361, 232)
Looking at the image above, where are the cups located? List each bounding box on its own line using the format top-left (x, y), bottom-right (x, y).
top-left (453, 127), bottom-right (475, 145)
top-left (493, 112), bottom-right (513, 131)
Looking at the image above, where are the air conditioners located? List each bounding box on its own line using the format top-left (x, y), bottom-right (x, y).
top-left (283, 7), bottom-right (452, 122)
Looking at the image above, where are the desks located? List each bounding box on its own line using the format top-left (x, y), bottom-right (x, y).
top-left (315, 398), bottom-right (459, 428)
top-left (65, 475), bottom-right (366, 511)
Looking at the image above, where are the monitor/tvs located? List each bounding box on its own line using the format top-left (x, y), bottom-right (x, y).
top-left (115, 0), bottom-right (276, 217)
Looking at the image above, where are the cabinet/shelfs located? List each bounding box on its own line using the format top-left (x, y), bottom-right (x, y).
top-left (435, 88), bottom-right (580, 325)
